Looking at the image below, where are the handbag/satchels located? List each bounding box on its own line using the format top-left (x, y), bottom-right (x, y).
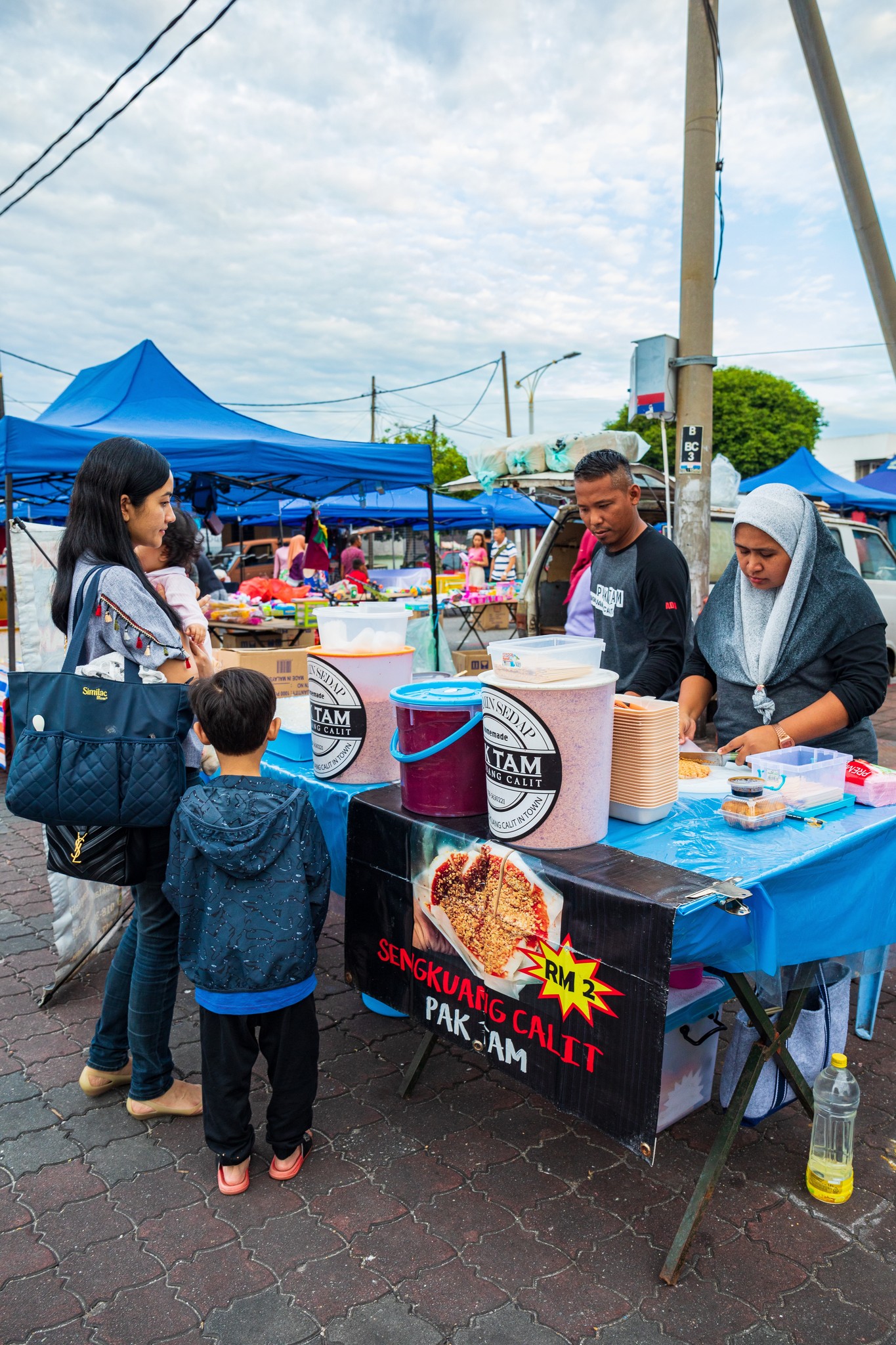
top-left (4, 670), bottom-right (194, 829)
top-left (41, 818), bottom-right (152, 888)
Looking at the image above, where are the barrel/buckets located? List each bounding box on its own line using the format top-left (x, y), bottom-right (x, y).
top-left (304, 644), bottom-right (416, 785)
top-left (669, 962), bottom-right (704, 989)
top-left (388, 679), bottom-right (488, 818)
top-left (476, 668), bottom-right (619, 851)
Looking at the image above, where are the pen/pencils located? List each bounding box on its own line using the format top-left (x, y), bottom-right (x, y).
top-left (786, 814), bottom-right (823, 825)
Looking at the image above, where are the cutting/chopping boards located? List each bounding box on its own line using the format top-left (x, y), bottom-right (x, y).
top-left (678, 761), bottom-right (753, 793)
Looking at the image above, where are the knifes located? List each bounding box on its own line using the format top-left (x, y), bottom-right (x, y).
top-left (679, 752), bottom-right (739, 766)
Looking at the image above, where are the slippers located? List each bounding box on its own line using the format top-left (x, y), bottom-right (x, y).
top-left (215, 1151), bottom-right (249, 1195)
top-left (268, 1130), bottom-right (313, 1181)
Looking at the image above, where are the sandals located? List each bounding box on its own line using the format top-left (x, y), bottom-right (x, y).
top-left (79, 1065), bottom-right (133, 1097)
top-left (126, 1083), bottom-right (203, 1120)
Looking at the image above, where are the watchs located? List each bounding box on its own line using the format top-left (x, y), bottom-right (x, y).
top-left (774, 722), bottom-right (797, 750)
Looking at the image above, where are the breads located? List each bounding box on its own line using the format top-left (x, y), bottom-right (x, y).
top-left (722, 799), bottom-right (787, 828)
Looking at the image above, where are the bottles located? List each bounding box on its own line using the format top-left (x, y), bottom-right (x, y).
top-left (805, 1052), bottom-right (861, 1205)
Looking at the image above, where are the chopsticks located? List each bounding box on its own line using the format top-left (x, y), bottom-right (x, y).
top-left (765, 776), bottom-right (844, 811)
top-left (493, 655), bottom-right (597, 683)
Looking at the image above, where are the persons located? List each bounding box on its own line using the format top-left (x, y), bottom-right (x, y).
top-left (274, 535), bottom-right (309, 587)
top-left (51, 436), bottom-right (210, 1122)
top-left (130, 504), bottom-right (215, 659)
top-left (422, 538), bottom-right (442, 575)
top-left (565, 526), bottom-right (600, 639)
top-left (573, 450), bottom-right (694, 703)
top-left (159, 667), bottom-right (334, 1195)
top-left (482, 529), bottom-right (493, 582)
top-left (340, 533), bottom-right (370, 580)
top-left (347, 559), bottom-right (368, 594)
top-left (672, 484), bottom-right (889, 767)
top-left (489, 526), bottom-right (519, 623)
top-left (461, 532), bottom-right (489, 622)
top-left (183, 546), bottom-right (229, 600)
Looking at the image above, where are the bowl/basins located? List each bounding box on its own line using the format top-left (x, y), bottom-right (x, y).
top-left (714, 789), bottom-right (794, 832)
top-left (486, 632), bottom-right (607, 683)
top-left (609, 693), bottom-right (679, 826)
top-left (726, 776), bottom-right (768, 797)
top-left (312, 602), bottom-right (415, 654)
top-left (413, 841), bottom-right (563, 994)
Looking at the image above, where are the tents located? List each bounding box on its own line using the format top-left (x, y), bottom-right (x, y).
top-left (236, 485), bottom-right (495, 593)
top-left (736, 446), bottom-right (896, 526)
top-left (0, 338), bottom-right (442, 748)
top-left (412, 490), bottom-right (591, 571)
top-left (856, 453), bottom-right (896, 495)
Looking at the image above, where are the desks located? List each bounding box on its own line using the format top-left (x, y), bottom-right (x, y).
top-left (200, 739), bottom-right (896, 1287)
top-left (336, 593), bottom-right (418, 603)
top-left (408, 600), bottom-right (520, 652)
top-left (207, 615), bottom-right (314, 647)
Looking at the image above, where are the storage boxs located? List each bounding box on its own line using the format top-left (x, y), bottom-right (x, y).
top-left (451, 579), bottom-right (607, 683)
top-left (206, 595), bottom-right (337, 763)
top-left (745, 746), bottom-right (854, 810)
top-left (655, 972), bottom-right (735, 1134)
